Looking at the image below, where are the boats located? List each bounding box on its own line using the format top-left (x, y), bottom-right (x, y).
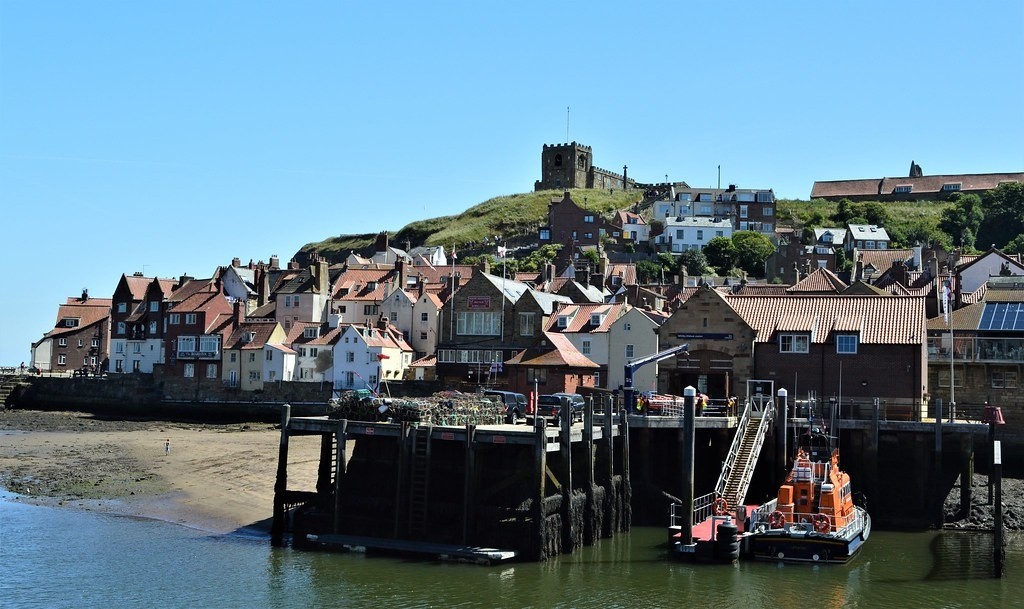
top-left (741, 387), bottom-right (872, 565)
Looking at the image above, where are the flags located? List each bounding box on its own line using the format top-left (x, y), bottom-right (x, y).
top-left (451, 247), bottom-right (457, 259)
top-left (377, 354), bottom-right (390, 362)
top-left (497, 246), bottom-right (506, 257)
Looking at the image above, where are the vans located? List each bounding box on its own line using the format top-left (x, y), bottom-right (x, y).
top-left (552, 393), bottom-right (586, 426)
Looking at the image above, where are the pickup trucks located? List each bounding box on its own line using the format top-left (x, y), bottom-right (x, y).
top-left (481, 390), bottom-right (529, 425)
top-left (524, 394), bottom-right (577, 427)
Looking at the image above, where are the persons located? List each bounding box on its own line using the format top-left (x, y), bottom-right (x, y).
top-left (610, 188), bottom-right (613, 194)
top-left (636, 393), bottom-right (651, 416)
top-left (20, 362), bottom-right (26, 372)
top-left (696, 389), bottom-right (737, 416)
top-left (464, 234), bottom-right (503, 249)
top-left (84, 364), bottom-right (99, 375)
top-left (629, 187), bottom-right (670, 215)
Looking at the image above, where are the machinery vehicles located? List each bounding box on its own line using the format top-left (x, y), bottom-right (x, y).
top-left (623, 342), bottom-right (691, 416)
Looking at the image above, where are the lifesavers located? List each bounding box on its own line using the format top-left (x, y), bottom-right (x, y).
top-left (769, 511), bottom-right (785, 528)
top-left (813, 513), bottom-right (829, 530)
top-left (712, 498), bottom-right (728, 515)
